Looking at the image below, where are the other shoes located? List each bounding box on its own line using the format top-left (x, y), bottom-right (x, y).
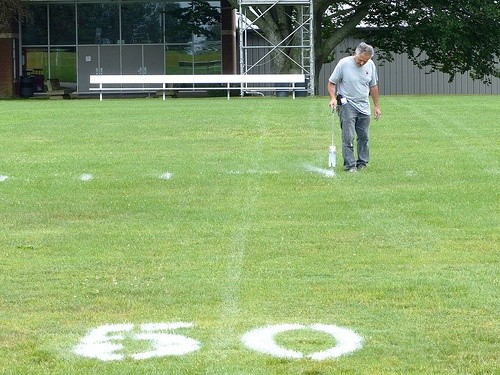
top-left (348, 167), bottom-right (356, 172)
top-left (357, 163), bottom-right (366, 169)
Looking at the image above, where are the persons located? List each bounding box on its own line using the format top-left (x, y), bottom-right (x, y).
top-left (327, 41), bottom-right (381, 174)
